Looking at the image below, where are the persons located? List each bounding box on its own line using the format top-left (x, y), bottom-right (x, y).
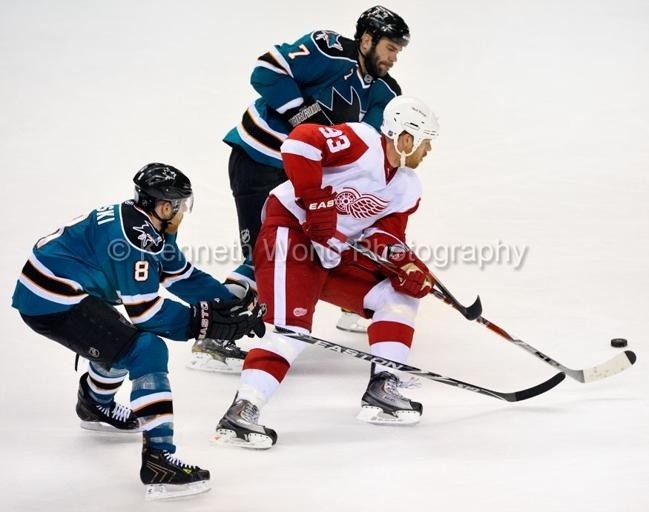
top-left (11, 158), bottom-right (271, 490)
top-left (214, 92), bottom-right (443, 448)
top-left (186, 0), bottom-right (413, 369)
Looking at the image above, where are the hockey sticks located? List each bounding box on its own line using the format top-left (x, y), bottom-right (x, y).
top-left (345, 241), bottom-right (636, 381)
top-left (263, 323), bottom-right (566, 403)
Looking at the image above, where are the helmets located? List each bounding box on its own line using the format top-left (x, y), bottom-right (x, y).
top-left (131, 162), bottom-right (195, 217)
top-left (380, 95), bottom-right (442, 144)
top-left (356, 5), bottom-right (411, 48)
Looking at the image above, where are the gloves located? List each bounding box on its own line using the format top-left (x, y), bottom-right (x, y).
top-left (377, 252), bottom-right (434, 298)
top-left (295, 186), bottom-right (339, 247)
top-left (187, 299), bottom-right (267, 344)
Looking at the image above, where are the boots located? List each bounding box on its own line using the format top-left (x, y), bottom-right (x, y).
top-left (191, 338), bottom-right (250, 359)
top-left (336, 308), bottom-right (371, 333)
top-left (225, 279), bottom-right (266, 339)
top-left (215, 390), bottom-right (277, 445)
top-left (361, 362), bottom-right (423, 414)
top-left (74, 372), bottom-right (139, 429)
top-left (139, 430), bottom-right (209, 484)
